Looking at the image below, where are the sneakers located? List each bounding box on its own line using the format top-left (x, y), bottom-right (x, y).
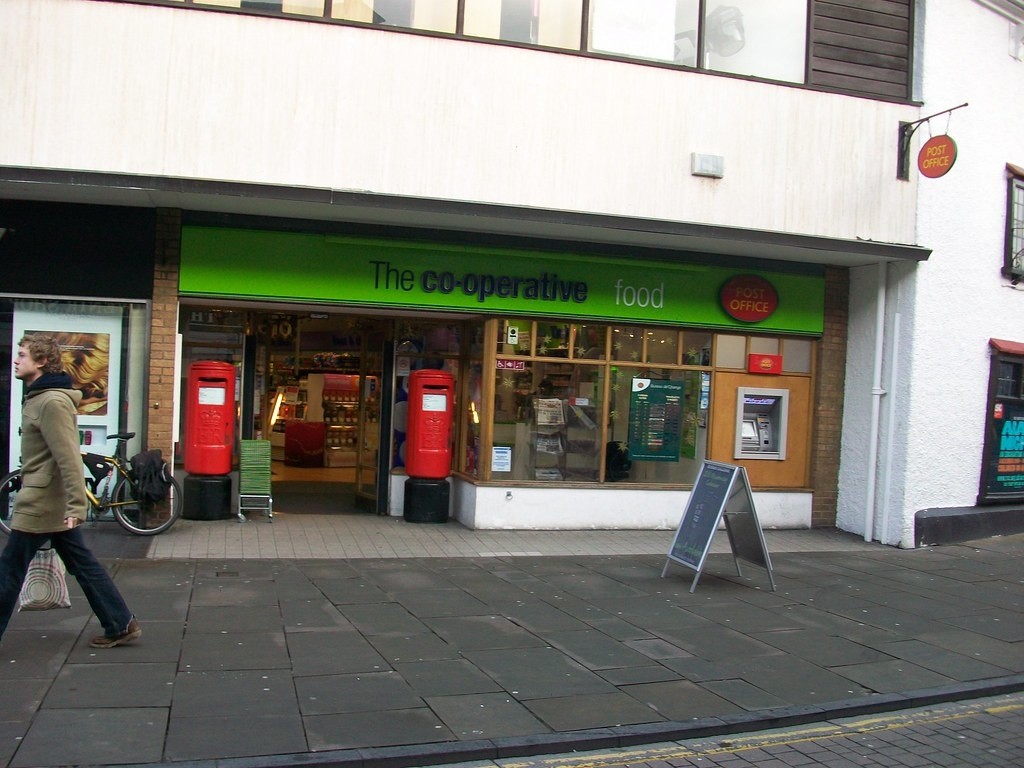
top-left (90, 615), bottom-right (142, 648)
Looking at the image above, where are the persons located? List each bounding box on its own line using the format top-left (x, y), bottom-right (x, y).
top-left (0, 335), bottom-right (142, 649)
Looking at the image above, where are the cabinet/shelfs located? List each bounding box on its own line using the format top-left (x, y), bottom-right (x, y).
top-left (308, 373), bottom-right (379, 467)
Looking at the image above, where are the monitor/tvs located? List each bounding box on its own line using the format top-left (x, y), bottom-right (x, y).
top-left (742, 422), bottom-right (754, 437)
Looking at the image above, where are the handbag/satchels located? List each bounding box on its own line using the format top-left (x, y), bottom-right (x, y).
top-left (18, 540), bottom-right (71, 613)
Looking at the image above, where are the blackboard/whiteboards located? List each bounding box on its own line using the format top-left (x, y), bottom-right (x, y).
top-left (667, 459), bottom-right (774, 571)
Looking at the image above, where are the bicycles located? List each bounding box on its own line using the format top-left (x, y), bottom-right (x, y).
top-left (0, 431), bottom-right (183, 539)
